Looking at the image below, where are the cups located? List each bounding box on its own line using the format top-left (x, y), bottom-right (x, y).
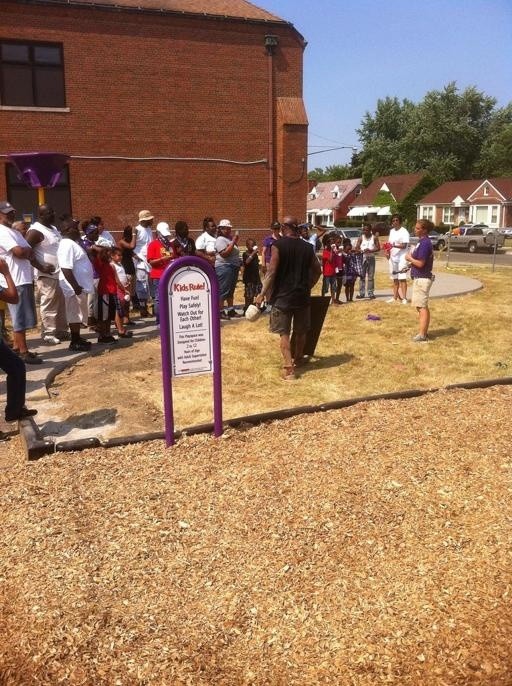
top-left (243, 304), bottom-right (261, 321)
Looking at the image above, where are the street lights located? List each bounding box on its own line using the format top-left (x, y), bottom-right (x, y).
top-left (307, 146), bottom-right (360, 156)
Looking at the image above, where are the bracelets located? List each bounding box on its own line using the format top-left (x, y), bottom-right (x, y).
top-left (410, 259), bottom-right (415, 263)
top-left (249, 255), bottom-right (254, 260)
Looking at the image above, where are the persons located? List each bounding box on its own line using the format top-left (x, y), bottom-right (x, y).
top-left (242, 237), bottom-right (267, 314)
top-left (384, 212), bottom-right (411, 304)
top-left (196, 216), bottom-right (243, 319)
top-left (0, 198), bottom-right (178, 366)
top-left (172, 221), bottom-right (195, 256)
top-left (405, 218), bottom-right (437, 341)
top-left (0, 259), bottom-right (39, 422)
top-left (261, 219), bottom-right (382, 305)
top-left (256, 213), bottom-right (322, 380)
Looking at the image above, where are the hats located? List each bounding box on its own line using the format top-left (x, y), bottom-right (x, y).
top-left (218, 218), bottom-right (234, 229)
top-left (157, 222), bottom-right (172, 237)
top-left (59, 218), bottom-right (80, 230)
top-left (271, 220), bottom-right (281, 229)
top-left (0, 201), bottom-right (17, 214)
top-left (138, 209), bottom-right (155, 221)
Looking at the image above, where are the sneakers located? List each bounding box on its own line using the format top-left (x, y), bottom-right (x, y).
top-left (18, 351), bottom-right (43, 365)
top-left (12, 348), bottom-right (20, 357)
top-left (5, 405), bottom-right (37, 422)
top-left (331, 291), bottom-right (408, 305)
top-left (88, 306), bottom-right (159, 345)
top-left (69, 337), bottom-right (92, 352)
top-left (44, 333), bottom-right (61, 345)
top-left (0, 431), bottom-right (9, 442)
top-left (228, 309), bottom-right (241, 318)
top-left (412, 334), bottom-right (429, 341)
top-left (55, 331), bottom-right (72, 341)
top-left (219, 310), bottom-right (231, 320)
top-left (281, 367), bottom-right (299, 380)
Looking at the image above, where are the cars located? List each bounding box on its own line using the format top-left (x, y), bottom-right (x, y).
top-left (320, 223), bottom-right (361, 250)
top-left (360, 220), bottom-right (390, 236)
top-left (406, 223), bottom-right (512, 253)
